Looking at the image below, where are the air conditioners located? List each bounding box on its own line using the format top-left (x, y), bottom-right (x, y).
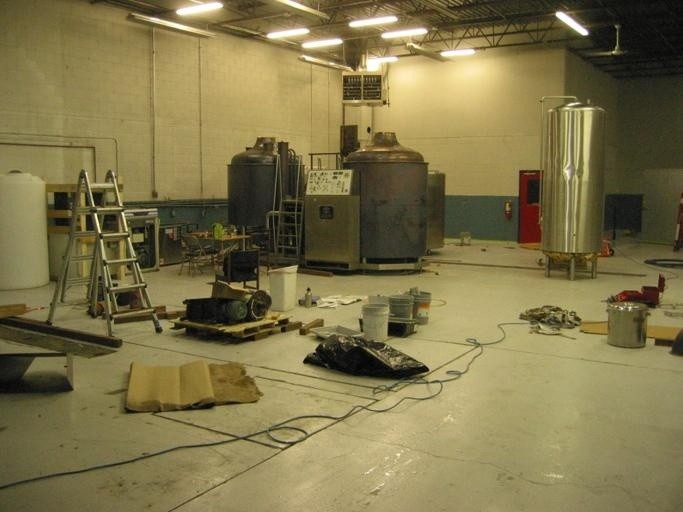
top-left (339, 71), bottom-right (387, 106)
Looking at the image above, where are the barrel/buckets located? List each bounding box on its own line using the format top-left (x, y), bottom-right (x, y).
top-left (359, 302), bottom-right (390, 342)
top-left (405, 289), bottom-right (432, 326)
top-left (0, 169), bottom-right (52, 290)
top-left (388, 295), bottom-right (416, 322)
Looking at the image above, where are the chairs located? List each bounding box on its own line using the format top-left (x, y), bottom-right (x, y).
top-left (178, 224), bottom-right (262, 291)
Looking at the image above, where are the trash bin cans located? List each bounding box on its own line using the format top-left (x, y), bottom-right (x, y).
top-left (267, 264), bottom-right (298, 312)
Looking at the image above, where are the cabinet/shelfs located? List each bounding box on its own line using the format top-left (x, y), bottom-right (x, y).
top-left (43, 181), bottom-right (129, 285)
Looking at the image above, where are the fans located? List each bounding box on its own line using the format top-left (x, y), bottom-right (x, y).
top-left (583, 23), bottom-right (659, 57)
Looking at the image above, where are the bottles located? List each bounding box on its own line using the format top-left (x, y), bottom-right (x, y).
top-left (305, 287), bottom-right (312, 308)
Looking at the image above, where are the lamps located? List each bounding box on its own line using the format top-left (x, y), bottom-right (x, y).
top-left (174, 1), bottom-right (224, 17)
top-left (440, 47), bottom-right (476, 58)
top-left (381, 27), bottom-right (430, 39)
top-left (366, 55), bottom-right (400, 62)
top-left (348, 15), bottom-right (401, 28)
top-left (267, 27), bottom-right (311, 40)
top-left (302, 38), bottom-right (343, 50)
top-left (553, 6), bottom-right (589, 37)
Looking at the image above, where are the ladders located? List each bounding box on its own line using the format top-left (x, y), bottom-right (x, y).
top-left (45, 170), bottom-right (163, 335)
